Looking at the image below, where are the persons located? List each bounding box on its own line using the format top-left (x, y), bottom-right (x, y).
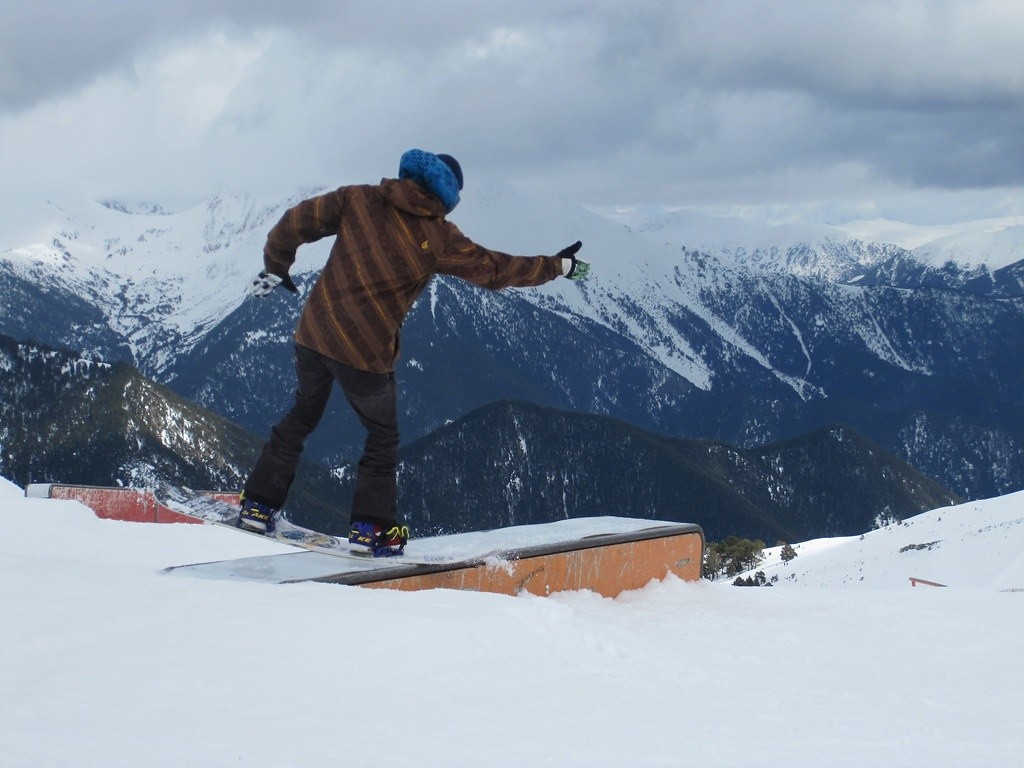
top-left (239, 153), bottom-right (591, 558)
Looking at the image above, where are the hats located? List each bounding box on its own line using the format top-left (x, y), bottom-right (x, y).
top-left (435, 154), bottom-right (463, 190)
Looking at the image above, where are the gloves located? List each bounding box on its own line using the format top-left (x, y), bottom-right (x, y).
top-left (556, 240), bottom-right (591, 280)
top-left (253, 269), bottom-right (297, 297)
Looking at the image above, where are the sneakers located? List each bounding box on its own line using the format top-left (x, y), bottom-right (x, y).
top-left (236, 489), bottom-right (277, 538)
top-left (349, 520), bottom-right (408, 558)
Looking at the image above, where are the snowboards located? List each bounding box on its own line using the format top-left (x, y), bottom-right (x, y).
top-left (149, 486), bottom-right (497, 564)
